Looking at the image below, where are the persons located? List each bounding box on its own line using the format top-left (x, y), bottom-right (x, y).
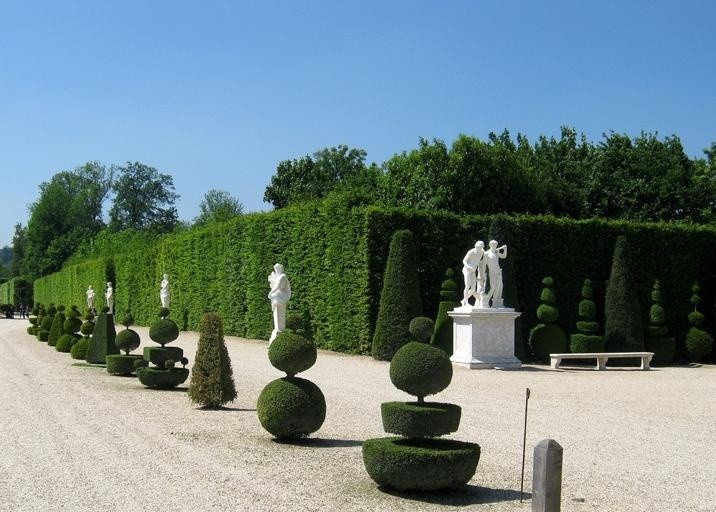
top-left (20, 304), bottom-right (30, 319)
top-left (268, 263), bottom-right (291, 331)
top-left (86, 285), bottom-right (95, 308)
top-left (105, 282), bottom-right (113, 314)
top-left (160, 274), bottom-right (170, 319)
top-left (461, 240), bottom-right (507, 308)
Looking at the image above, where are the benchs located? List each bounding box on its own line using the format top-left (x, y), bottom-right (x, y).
top-left (549, 352), bottom-right (654, 371)
